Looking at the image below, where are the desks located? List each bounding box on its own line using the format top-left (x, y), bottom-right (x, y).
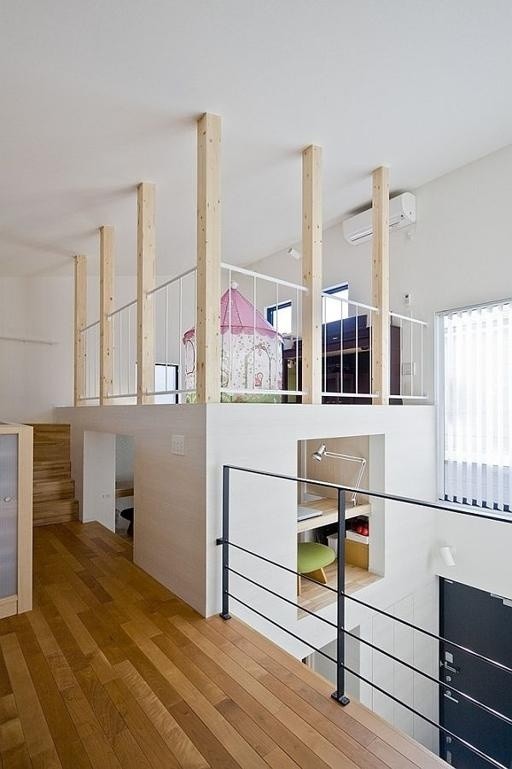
top-left (298, 495), bottom-right (370, 534)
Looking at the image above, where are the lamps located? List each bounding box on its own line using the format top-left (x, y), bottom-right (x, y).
top-left (312, 445), bottom-right (366, 506)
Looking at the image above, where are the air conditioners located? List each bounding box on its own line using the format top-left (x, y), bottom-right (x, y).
top-left (341, 192), bottom-right (416, 245)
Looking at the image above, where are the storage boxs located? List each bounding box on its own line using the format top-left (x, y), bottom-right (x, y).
top-left (327, 533), bottom-right (338, 556)
top-left (346, 539), bottom-right (368, 570)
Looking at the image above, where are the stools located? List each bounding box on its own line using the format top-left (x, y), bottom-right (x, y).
top-left (121, 508), bottom-right (134, 535)
top-left (297, 542), bottom-right (336, 596)
